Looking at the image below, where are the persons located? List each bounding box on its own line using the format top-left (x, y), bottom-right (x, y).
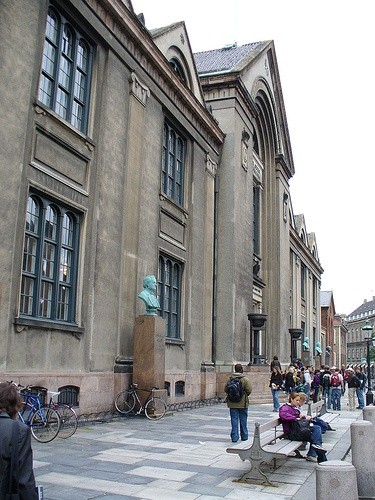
top-left (279, 393), bottom-right (328, 463)
top-left (303, 338), bottom-right (333, 356)
top-left (282, 354), bottom-right (332, 404)
top-left (269, 366), bottom-right (284, 412)
top-left (330, 368), bottom-right (344, 411)
top-left (355, 367), bottom-right (367, 409)
top-left (137, 274), bottom-right (161, 309)
top-left (224, 364), bottom-right (252, 443)
top-left (295, 392), bottom-right (324, 462)
top-left (344, 367), bottom-right (361, 411)
top-left (0, 381), bottom-right (35, 500)
top-left (271, 355), bottom-right (281, 372)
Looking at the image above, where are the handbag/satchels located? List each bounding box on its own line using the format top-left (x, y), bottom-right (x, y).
top-left (312, 416), bottom-right (328, 433)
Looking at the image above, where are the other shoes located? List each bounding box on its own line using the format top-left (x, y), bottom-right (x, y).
top-left (307, 455), bottom-right (317, 462)
top-left (356, 406), bottom-right (361, 409)
top-left (311, 444), bottom-right (327, 453)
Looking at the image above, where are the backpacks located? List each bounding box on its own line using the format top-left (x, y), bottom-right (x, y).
top-left (352, 375), bottom-right (361, 387)
top-left (288, 419), bottom-right (311, 442)
top-left (330, 373), bottom-right (340, 387)
top-left (225, 376), bottom-right (244, 402)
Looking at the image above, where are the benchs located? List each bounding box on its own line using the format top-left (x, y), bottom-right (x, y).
top-left (226, 400), bottom-right (340, 487)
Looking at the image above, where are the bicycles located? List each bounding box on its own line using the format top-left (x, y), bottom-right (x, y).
top-left (25, 384), bottom-right (78, 439)
top-left (10, 379), bottom-right (62, 443)
top-left (114, 382), bottom-right (166, 419)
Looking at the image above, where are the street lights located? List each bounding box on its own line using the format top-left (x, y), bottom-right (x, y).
top-left (362, 321), bottom-right (374, 405)
